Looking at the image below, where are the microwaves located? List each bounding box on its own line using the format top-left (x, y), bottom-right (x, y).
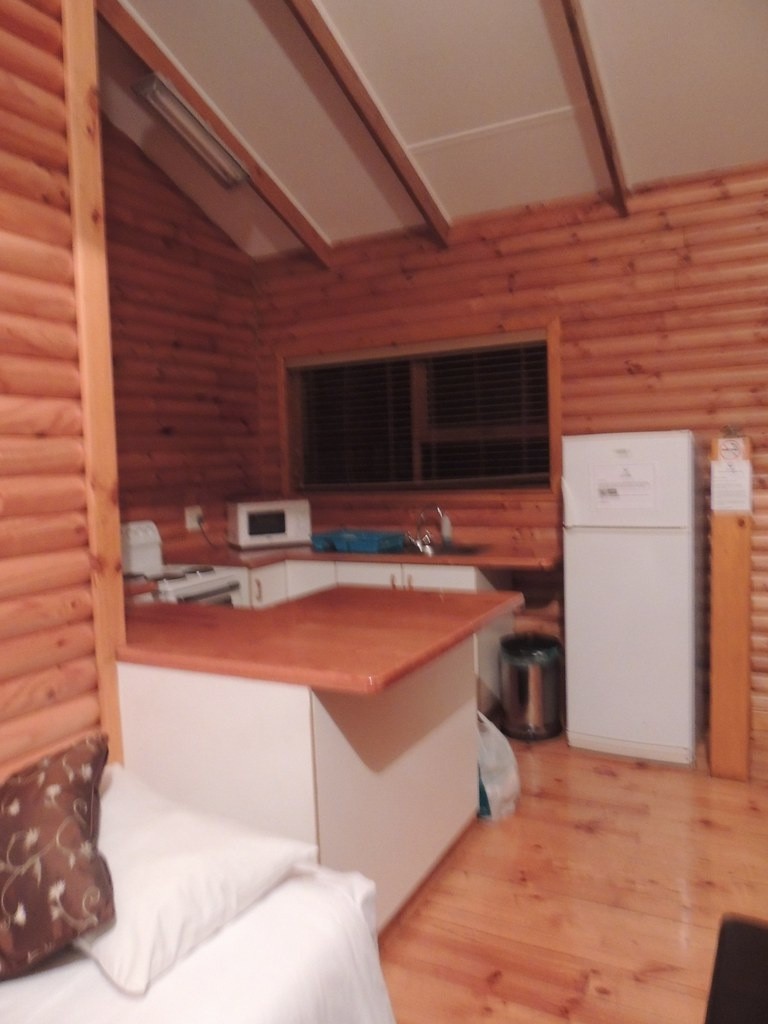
top-left (226, 497), bottom-right (312, 550)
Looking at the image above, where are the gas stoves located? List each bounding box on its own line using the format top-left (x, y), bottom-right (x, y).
top-left (119, 519), bottom-right (250, 609)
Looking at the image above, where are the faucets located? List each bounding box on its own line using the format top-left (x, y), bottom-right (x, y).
top-left (407, 503), bottom-right (445, 553)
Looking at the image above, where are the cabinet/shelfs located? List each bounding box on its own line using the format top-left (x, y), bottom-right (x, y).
top-left (165, 541), bottom-right (554, 715)
top-left (116, 581), bottom-right (525, 933)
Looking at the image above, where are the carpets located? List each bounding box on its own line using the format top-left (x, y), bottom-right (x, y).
top-left (704, 910), bottom-right (767, 1024)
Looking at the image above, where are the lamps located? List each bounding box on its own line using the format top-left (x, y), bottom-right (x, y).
top-left (131, 72), bottom-right (250, 190)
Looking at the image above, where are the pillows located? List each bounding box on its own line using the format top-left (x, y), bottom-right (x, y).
top-left (0, 734), bottom-right (115, 981)
top-left (70, 760), bottom-right (317, 997)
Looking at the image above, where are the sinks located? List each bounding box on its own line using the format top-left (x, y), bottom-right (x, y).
top-left (385, 540), bottom-right (483, 559)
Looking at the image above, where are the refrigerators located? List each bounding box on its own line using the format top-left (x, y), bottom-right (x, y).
top-left (559, 429), bottom-right (707, 765)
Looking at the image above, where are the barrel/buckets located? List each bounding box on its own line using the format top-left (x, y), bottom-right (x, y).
top-left (498, 632), bottom-right (560, 739)
